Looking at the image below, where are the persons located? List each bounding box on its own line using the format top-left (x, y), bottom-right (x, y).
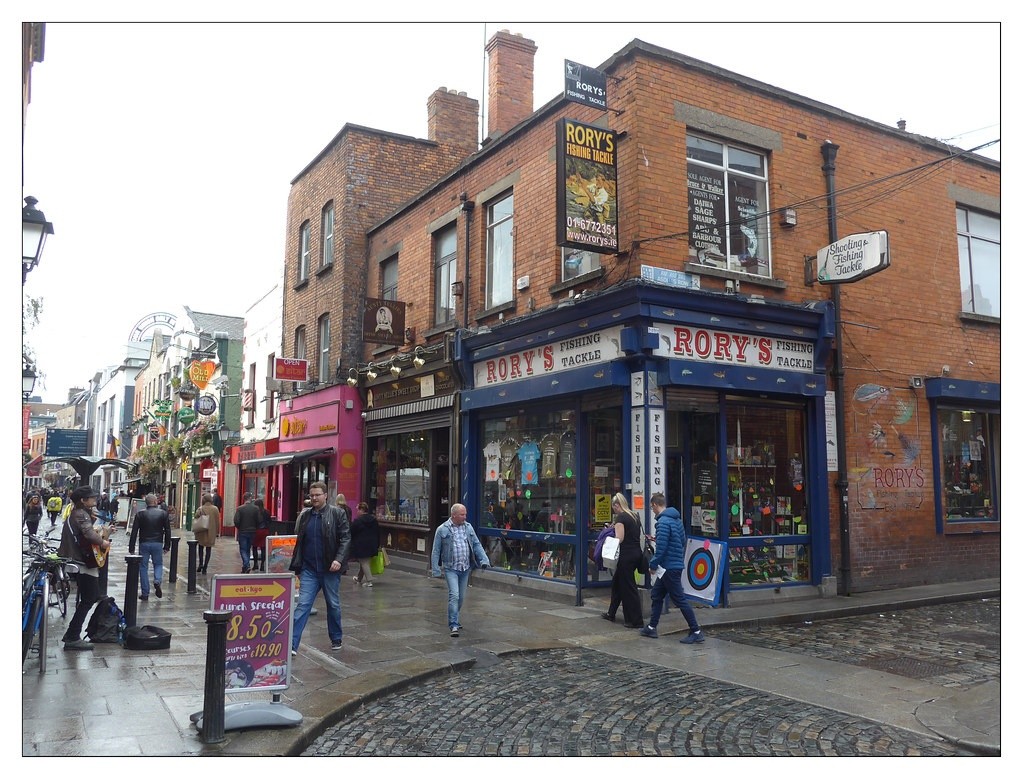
top-left (294, 499), bottom-right (312, 536)
top-left (22, 484), bottom-right (120, 546)
top-left (430, 502), bottom-right (490, 637)
top-left (287, 482), bottom-right (351, 656)
top-left (56, 485), bottom-right (110, 650)
top-left (639, 493), bottom-right (705, 644)
top-left (350, 501), bottom-right (383, 588)
top-left (129, 494), bottom-right (171, 601)
top-left (234, 491), bottom-right (258, 574)
top-left (252, 498), bottom-right (277, 572)
top-left (193, 493), bottom-right (221, 574)
top-left (211, 488), bottom-right (222, 513)
top-left (334, 493), bottom-right (352, 575)
top-left (600, 492), bottom-right (644, 628)
top-left (507, 497), bottom-right (553, 566)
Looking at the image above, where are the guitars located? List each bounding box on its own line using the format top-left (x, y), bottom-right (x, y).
top-left (78, 518), bottom-right (119, 569)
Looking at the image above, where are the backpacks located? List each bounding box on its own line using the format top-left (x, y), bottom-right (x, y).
top-left (85, 598), bottom-right (123, 642)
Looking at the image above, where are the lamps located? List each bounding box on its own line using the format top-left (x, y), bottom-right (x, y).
top-left (413, 345), bottom-right (436, 370)
top-left (367, 360), bottom-right (386, 382)
top-left (908, 376), bottom-right (924, 389)
top-left (347, 368), bottom-right (366, 388)
top-left (390, 354), bottom-right (408, 378)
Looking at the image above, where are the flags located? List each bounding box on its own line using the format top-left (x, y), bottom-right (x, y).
top-left (105, 408), bottom-right (166, 459)
top-left (27, 455), bottom-right (43, 477)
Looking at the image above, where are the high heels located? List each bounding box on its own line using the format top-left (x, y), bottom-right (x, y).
top-left (197, 564), bottom-right (204, 572)
top-left (202, 567), bottom-right (207, 574)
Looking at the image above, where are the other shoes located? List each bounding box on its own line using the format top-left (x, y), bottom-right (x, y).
top-left (450, 627), bottom-right (459, 636)
top-left (252, 566), bottom-right (259, 570)
top-left (260, 565), bottom-right (264, 571)
top-left (244, 568), bottom-right (251, 573)
top-left (458, 622), bottom-right (462, 627)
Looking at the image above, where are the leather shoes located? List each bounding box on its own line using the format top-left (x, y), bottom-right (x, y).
top-left (139, 595), bottom-right (148, 601)
top-left (154, 582), bottom-right (162, 598)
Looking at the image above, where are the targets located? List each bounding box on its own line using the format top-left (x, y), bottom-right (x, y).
top-left (680, 537), bottom-right (727, 608)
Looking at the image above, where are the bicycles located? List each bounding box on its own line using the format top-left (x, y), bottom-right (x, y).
top-left (22, 528), bottom-right (71, 675)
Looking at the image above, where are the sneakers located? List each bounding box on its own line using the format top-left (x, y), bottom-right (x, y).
top-left (64, 639), bottom-right (94, 650)
top-left (363, 583), bottom-right (373, 587)
top-left (680, 631), bottom-right (705, 643)
top-left (639, 624), bottom-right (657, 638)
top-left (601, 612), bottom-right (615, 621)
top-left (623, 621), bottom-right (643, 628)
top-left (331, 639), bottom-right (342, 649)
top-left (292, 648), bottom-right (297, 656)
top-left (353, 576), bottom-right (361, 584)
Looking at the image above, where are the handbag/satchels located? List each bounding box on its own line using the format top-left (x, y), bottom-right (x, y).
top-left (369, 552), bottom-right (384, 574)
top-left (123, 626), bottom-right (172, 650)
top-left (192, 505), bottom-right (209, 532)
top-left (638, 543), bottom-right (654, 574)
top-left (602, 536), bottom-right (620, 569)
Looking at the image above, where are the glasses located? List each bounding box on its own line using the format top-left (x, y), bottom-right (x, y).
top-left (650, 505), bottom-right (656, 510)
top-left (307, 493), bottom-right (326, 498)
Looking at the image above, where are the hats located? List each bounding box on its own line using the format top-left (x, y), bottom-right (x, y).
top-left (335, 494), bottom-right (347, 504)
top-left (70, 485), bottom-right (98, 502)
